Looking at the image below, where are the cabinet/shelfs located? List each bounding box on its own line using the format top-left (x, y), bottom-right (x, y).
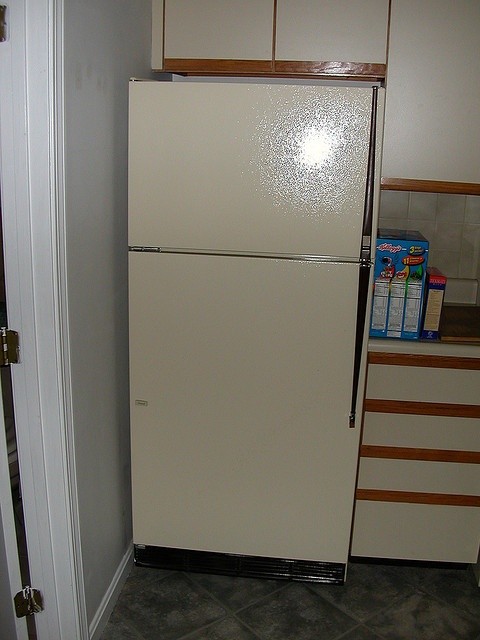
top-left (349, 341), bottom-right (480, 566)
top-left (150, 0), bottom-right (389, 78)
top-left (376, 0), bottom-right (480, 195)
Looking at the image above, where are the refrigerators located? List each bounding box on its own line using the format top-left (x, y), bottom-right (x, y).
top-left (126, 77), bottom-right (384, 586)
